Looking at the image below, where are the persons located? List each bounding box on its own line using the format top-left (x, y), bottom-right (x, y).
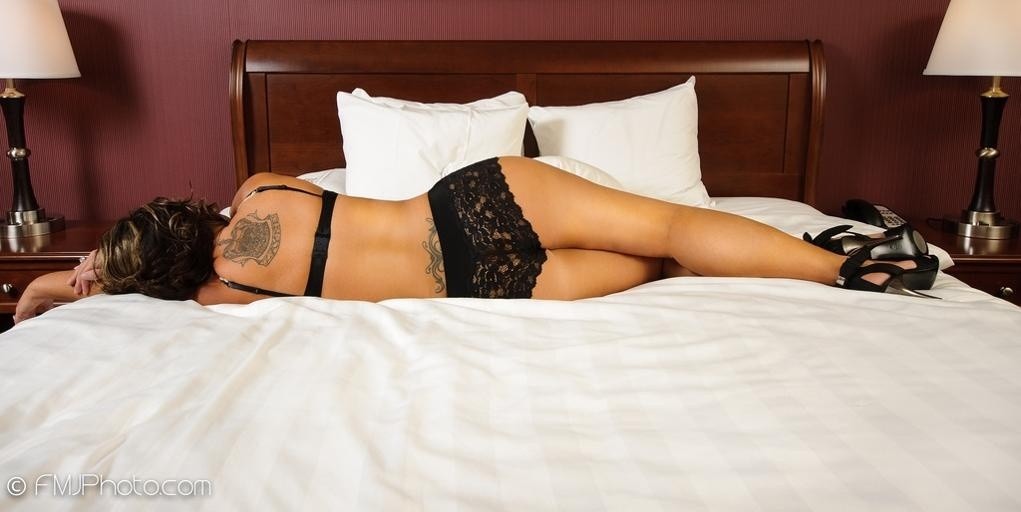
top-left (9, 156), bottom-right (946, 326)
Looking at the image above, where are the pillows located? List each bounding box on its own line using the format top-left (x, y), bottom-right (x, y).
top-left (334, 89), bottom-right (532, 196)
top-left (351, 87), bottom-right (526, 111)
top-left (523, 75), bottom-right (715, 211)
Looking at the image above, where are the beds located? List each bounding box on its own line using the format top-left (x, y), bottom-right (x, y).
top-left (3, 38), bottom-right (1018, 509)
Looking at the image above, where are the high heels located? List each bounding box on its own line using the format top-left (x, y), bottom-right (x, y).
top-left (835, 246), bottom-right (939, 298)
top-left (802, 222), bottom-right (928, 260)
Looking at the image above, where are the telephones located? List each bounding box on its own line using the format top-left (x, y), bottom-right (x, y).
top-left (843, 199), bottom-right (908, 230)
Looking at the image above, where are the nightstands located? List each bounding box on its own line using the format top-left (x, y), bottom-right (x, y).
top-left (898, 218), bottom-right (1020, 308)
top-left (0, 219), bottom-right (104, 328)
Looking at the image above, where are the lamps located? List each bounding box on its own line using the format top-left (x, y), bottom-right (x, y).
top-left (0, 0), bottom-right (84, 235)
top-left (915, 1), bottom-right (1020, 240)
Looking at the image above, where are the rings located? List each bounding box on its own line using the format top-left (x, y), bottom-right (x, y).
top-left (76, 249), bottom-right (88, 266)
